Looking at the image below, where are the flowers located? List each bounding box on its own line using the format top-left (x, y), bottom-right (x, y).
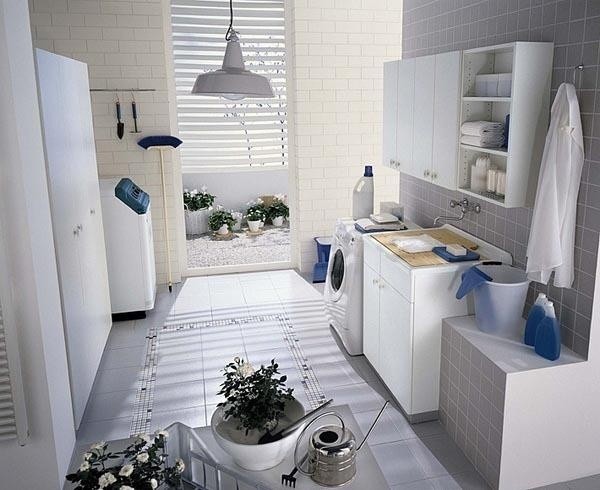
top-left (65, 431), bottom-right (186, 490)
top-left (207, 210), bottom-right (238, 231)
top-left (215, 354), bottom-right (298, 437)
top-left (245, 197), bottom-right (289, 220)
top-left (183, 185), bottom-right (213, 210)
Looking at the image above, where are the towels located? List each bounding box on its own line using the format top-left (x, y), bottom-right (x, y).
top-left (433, 245), bottom-right (480, 263)
top-left (355, 212), bottom-right (409, 232)
top-left (462, 120), bottom-right (505, 149)
top-left (389, 234), bottom-right (446, 254)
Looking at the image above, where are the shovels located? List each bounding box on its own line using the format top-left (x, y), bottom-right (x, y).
top-left (116, 102), bottom-right (124, 139)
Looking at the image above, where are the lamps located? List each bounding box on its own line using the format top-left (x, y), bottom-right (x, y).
top-left (189, 0), bottom-right (276, 103)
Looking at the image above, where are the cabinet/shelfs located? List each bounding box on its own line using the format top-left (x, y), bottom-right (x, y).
top-left (460, 41), bottom-right (554, 211)
top-left (363, 237), bottom-right (477, 426)
top-left (34, 47), bottom-right (116, 429)
top-left (416, 51), bottom-right (462, 192)
top-left (382, 57), bottom-right (415, 179)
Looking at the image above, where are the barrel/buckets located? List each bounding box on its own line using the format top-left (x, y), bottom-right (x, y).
top-left (472, 264), bottom-right (531, 338)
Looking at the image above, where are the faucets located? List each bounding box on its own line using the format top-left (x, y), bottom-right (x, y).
top-left (434, 198), bottom-right (481, 227)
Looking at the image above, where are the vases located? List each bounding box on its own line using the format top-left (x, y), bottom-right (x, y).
top-left (217, 224), bottom-right (229, 235)
top-left (212, 398), bottom-right (305, 473)
top-left (247, 217), bottom-right (286, 231)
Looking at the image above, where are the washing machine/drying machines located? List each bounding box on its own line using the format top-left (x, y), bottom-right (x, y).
top-left (98, 178), bottom-right (158, 313)
top-left (324, 217), bottom-right (423, 356)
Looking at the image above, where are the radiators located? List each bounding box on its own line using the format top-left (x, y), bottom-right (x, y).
top-left (0, 244), bottom-right (34, 450)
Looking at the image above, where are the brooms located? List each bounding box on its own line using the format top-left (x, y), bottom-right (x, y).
top-left (137, 135), bottom-right (183, 294)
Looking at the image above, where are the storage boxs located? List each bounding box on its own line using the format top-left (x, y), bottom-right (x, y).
top-left (474, 72), bottom-right (511, 97)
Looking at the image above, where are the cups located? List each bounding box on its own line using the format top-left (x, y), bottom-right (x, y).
top-left (486, 169), bottom-right (506, 197)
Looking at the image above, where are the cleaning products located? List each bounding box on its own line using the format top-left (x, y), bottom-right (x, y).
top-left (352, 165), bottom-right (374, 220)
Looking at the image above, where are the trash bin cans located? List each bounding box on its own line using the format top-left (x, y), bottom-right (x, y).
top-left (312, 235), bottom-right (333, 283)
